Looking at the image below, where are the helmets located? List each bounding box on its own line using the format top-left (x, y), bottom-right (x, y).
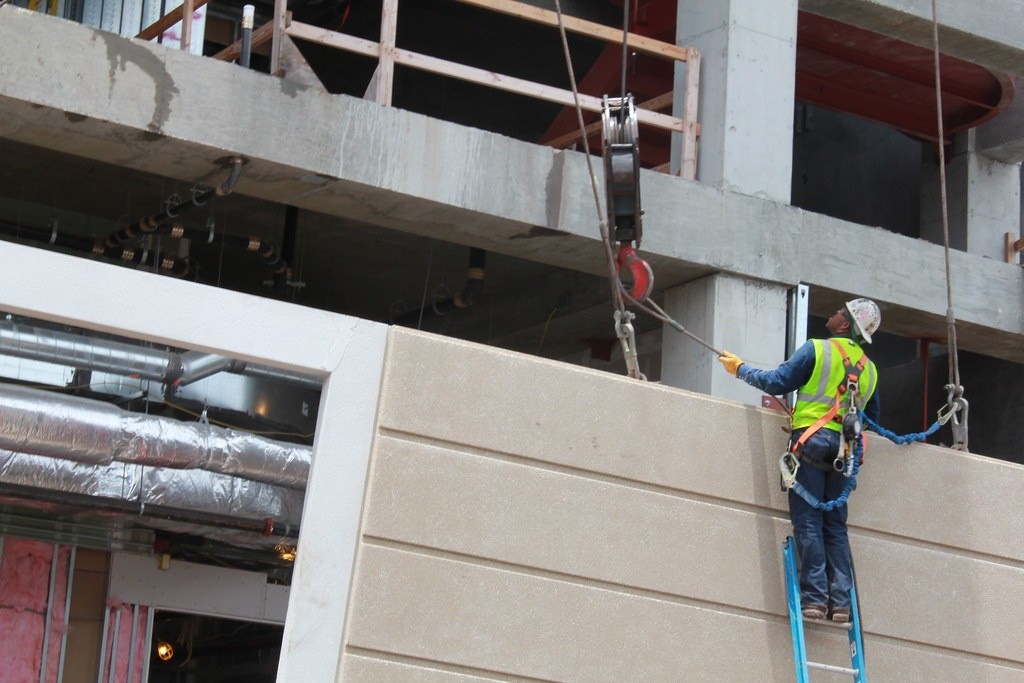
top-left (845, 298), bottom-right (882, 344)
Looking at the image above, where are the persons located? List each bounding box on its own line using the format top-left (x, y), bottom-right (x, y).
top-left (718, 297), bottom-right (882, 623)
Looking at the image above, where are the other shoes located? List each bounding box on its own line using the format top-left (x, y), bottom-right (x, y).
top-left (801, 604), bottom-right (851, 622)
